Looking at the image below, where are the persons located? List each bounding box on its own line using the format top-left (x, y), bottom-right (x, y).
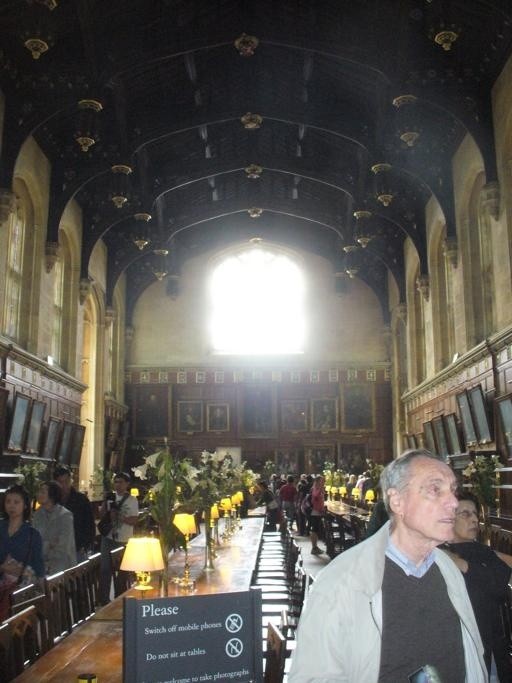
top-left (345, 468), bottom-right (379, 496)
top-left (309, 474), bottom-right (325, 554)
top-left (365, 499), bottom-right (388, 539)
top-left (53, 468), bottom-right (97, 622)
top-left (0, 483), bottom-right (46, 665)
top-left (98, 471), bottom-right (141, 606)
top-left (32, 479), bottom-right (79, 636)
top-left (285, 445), bottom-right (491, 682)
top-left (441, 489), bottom-right (512, 682)
top-left (253, 470), bottom-right (321, 537)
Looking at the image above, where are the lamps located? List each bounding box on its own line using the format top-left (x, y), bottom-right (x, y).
top-left (325, 486), bottom-right (376, 510)
top-left (207, 491), bottom-right (244, 550)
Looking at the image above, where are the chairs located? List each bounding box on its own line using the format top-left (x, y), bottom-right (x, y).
top-left (0, 510), bottom-right (512, 683)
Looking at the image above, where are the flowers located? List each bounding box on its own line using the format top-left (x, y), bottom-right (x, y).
top-left (461, 455), bottom-right (504, 509)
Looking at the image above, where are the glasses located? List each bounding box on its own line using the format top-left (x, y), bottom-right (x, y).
top-left (456, 511), bottom-right (478, 519)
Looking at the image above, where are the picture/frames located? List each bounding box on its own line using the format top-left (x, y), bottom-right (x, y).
top-left (131, 385), bottom-right (378, 476)
top-left (402, 383), bottom-right (512, 466)
top-left (0, 387), bottom-right (87, 477)
